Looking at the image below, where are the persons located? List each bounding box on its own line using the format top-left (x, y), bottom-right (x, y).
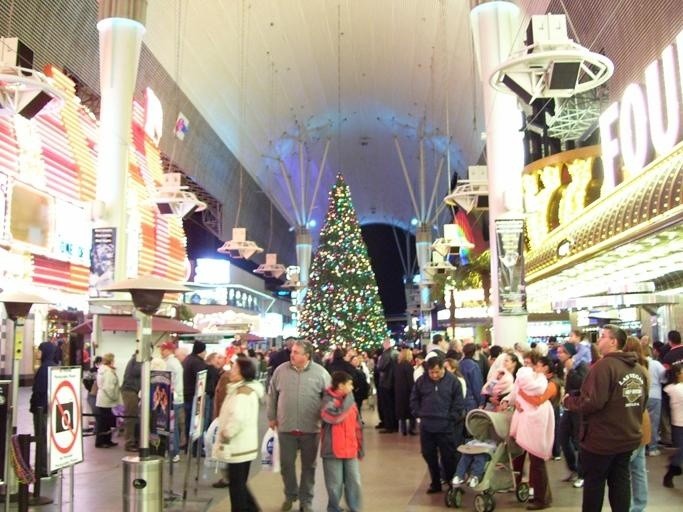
top-left (326, 348), bottom-right (359, 392)
top-left (409, 356), bottom-right (467, 495)
top-left (413, 354), bottom-right (425, 383)
top-left (653, 341), bottom-right (674, 447)
top-left (561, 323), bottom-right (651, 512)
top-left (266, 339), bottom-right (333, 512)
top-left (215, 354), bottom-right (227, 378)
top-left (622, 335), bottom-right (652, 512)
top-left (556, 342), bottom-right (591, 489)
top-left (514, 357), bottom-right (563, 510)
top-left (211, 352), bottom-right (246, 488)
top-left (349, 355), bottom-right (371, 425)
top-left (471, 343), bottom-right (488, 377)
top-left (553, 406), bottom-right (562, 461)
top-left (424, 334), bottom-right (447, 361)
top-left (480, 352), bottom-right (524, 413)
top-left (446, 339), bottom-right (463, 361)
top-left (29, 341), bottom-right (65, 478)
top-left (378, 337), bottom-right (400, 434)
top-left (545, 336), bottom-right (560, 361)
top-left (564, 329), bottom-right (593, 370)
top-left (451, 453), bottom-right (487, 489)
top-left (82, 355), bottom-right (103, 436)
top-left (439, 358), bottom-right (467, 486)
top-left (373, 353), bottom-right (384, 429)
top-left (210, 357), bottom-right (266, 512)
top-left (268, 336), bottom-right (298, 376)
top-left (119, 349), bottom-right (142, 452)
top-left (663, 363), bottom-right (683, 489)
top-left (662, 329), bottom-right (683, 383)
top-left (496, 372), bottom-right (505, 381)
top-left (200, 351), bottom-right (218, 442)
top-left (393, 348), bottom-right (419, 436)
top-left (94, 352), bottom-right (119, 449)
top-left (159, 340), bottom-right (185, 464)
top-left (320, 371), bottom-right (365, 512)
top-left (641, 342), bottom-right (666, 457)
top-left (180, 339), bottom-right (208, 458)
top-left (458, 342), bottom-right (486, 412)
top-left (497, 350), bottom-right (543, 496)
top-left (640, 335), bottom-right (653, 358)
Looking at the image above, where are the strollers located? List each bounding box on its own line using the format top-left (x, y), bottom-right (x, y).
top-left (445, 409), bottom-right (529, 512)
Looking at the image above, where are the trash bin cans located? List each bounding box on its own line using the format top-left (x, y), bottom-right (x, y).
top-left (122, 456), bottom-right (162, 511)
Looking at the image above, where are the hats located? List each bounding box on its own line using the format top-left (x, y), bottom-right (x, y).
top-left (191, 340), bottom-right (206, 354)
top-left (425, 351), bottom-right (437, 361)
top-left (157, 341), bottom-right (175, 349)
top-left (535, 342), bottom-right (548, 357)
top-left (562, 343), bottom-right (577, 356)
top-left (333, 345), bottom-right (347, 358)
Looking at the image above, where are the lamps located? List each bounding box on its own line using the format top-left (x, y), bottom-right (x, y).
top-left (253, 253), bottom-right (286, 279)
top-left (431, 237), bottom-right (455, 256)
top-left (145, 175), bottom-right (207, 219)
top-left (488, 14), bottom-right (614, 105)
top-left (443, 178), bottom-right (488, 212)
top-left (217, 229), bottom-right (263, 260)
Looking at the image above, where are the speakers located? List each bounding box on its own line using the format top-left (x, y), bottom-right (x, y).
top-left (451, 198), bottom-right (473, 215)
top-left (17, 89), bottom-right (52, 119)
top-left (157, 202), bottom-right (175, 217)
top-left (232, 227), bottom-right (249, 242)
top-left (466, 166), bottom-right (486, 181)
top-left (435, 269), bottom-right (446, 275)
top-left (476, 195), bottom-right (488, 210)
top-left (229, 249), bottom-right (241, 258)
top-left (1, 37), bottom-right (34, 76)
top-left (180, 203), bottom-right (198, 220)
top-left (544, 60), bottom-right (580, 95)
top-left (245, 250), bottom-right (257, 261)
top-left (525, 13), bottom-right (568, 54)
top-left (263, 270), bottom-right (272, 278)
top-left (430, 252), bottom-right (441, 265)
top-left (448, 246), bottom-right (459, 253)
top-left (266, 253), bottom-right (279, 265)
top-left (501, 74), bottom-right (533, 105)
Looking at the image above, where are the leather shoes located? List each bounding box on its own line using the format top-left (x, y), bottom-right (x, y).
top-left (299, 504), bottom-right (313, 512)
top-left (281, 495), bottom-right (298, 512)
top-left (426, 485), bottom-right (440, 493)
top-left (380, 429), bottom-right (392, 433)
top-left (393, 428), bottom-right (398, 431)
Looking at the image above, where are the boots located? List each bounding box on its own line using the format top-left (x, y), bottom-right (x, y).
top-left (663, 465), bottom-right (682, 488)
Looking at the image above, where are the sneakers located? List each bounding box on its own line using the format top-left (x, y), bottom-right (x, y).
top-left (567, 472), bottom-right (578, 482)
top-left (527, 502), bottom-right (547, 509)
top-left (165, 457), bottom-right (177, 462)
top-left (109, 441), bottom-right (119, 446)
top-left (649, 450), bottom-right (661, 456)
top-left (470, 477), bottom-right (478, 487)
top-left (528, 496), bottom-right (534, 503)
top-left (453, 476), bottom-right (464, 484)
top-left (96, 443), bottom-right (109, 448)
top-left (176, 454), bottom-right (180, 461)
top-left (573, 479), bottom-right (584, 487)
top-left (213, 480), bottom-right (229, 487)
